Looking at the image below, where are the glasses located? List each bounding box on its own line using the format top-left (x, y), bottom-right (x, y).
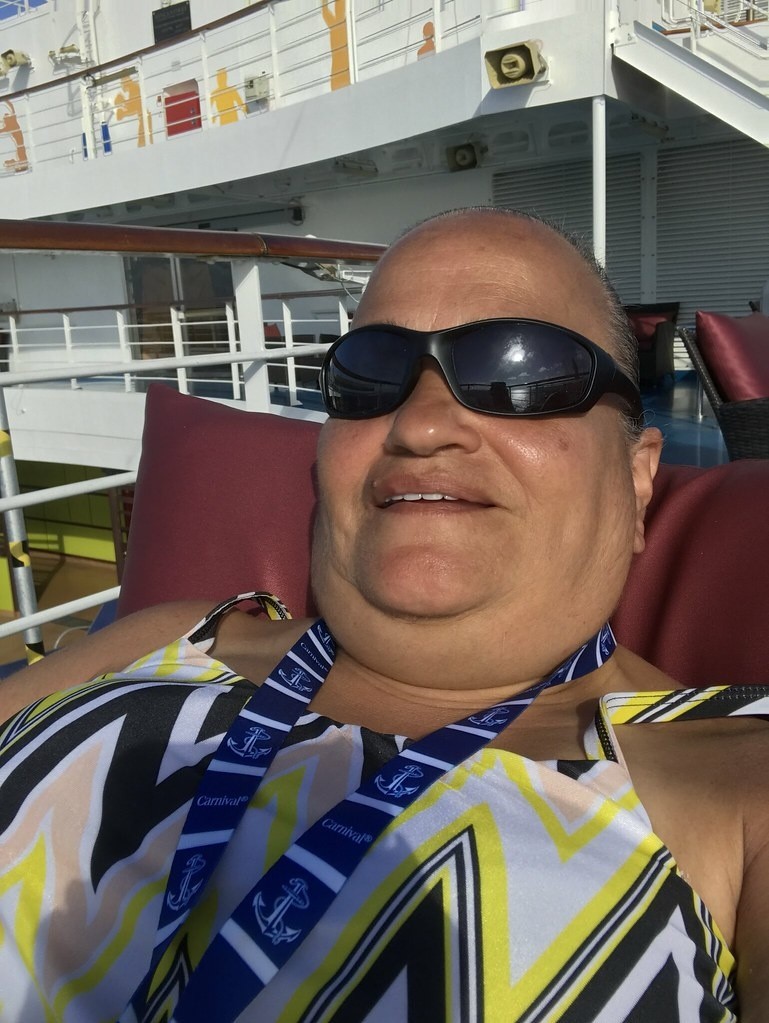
top-left (318, 317), bottom-right (647, 436)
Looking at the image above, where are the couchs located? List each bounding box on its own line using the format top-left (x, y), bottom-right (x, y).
top-left (110, 387), bottom-right (767, 689)
top-left (676, 309), bottom-right (769, 462)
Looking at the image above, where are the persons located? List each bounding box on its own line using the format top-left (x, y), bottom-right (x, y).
top-left (2, 209), bottom-right (769, 1022)
